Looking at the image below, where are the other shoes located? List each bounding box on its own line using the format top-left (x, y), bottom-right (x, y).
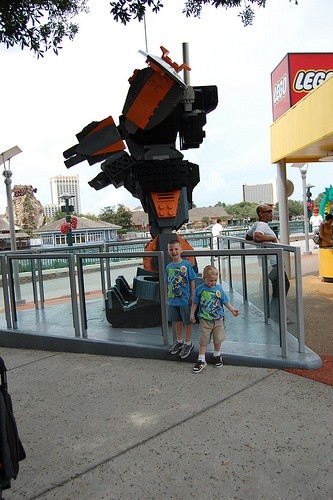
top-left (286, 317), bottom-right (295, 324)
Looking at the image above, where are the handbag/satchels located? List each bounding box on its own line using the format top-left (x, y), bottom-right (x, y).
top-left (312, 231), bottom-right (323, 245)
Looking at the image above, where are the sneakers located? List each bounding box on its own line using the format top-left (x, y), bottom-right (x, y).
top-left (168, 340), bottom-right (185, 355)
top-left (212, 353), bottom-right (223, 369)
top-left (178, 341), bottom-right (194, 359)
top-left (193, 359), bottom-right (207, 373)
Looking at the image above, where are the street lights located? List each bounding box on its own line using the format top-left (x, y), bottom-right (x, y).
top-left (59, 193), bottom-right (76, 246)
top-left (306, 184), bottom-right (316, 233)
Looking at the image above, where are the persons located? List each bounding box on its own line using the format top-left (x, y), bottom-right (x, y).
top-left (209, 219), bottom-right (229, 261)
top-left (310, 208), bottom-right (333, 279)
top-left (189, 265), bottom-right (239, 372)
top-left (164, 240), bottom-right (198, 359)
top-left (245, 205), bottom-right (289, 324)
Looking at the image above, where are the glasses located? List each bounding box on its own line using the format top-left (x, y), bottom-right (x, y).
top-left (260, 209), bottom-right (273, 214)
